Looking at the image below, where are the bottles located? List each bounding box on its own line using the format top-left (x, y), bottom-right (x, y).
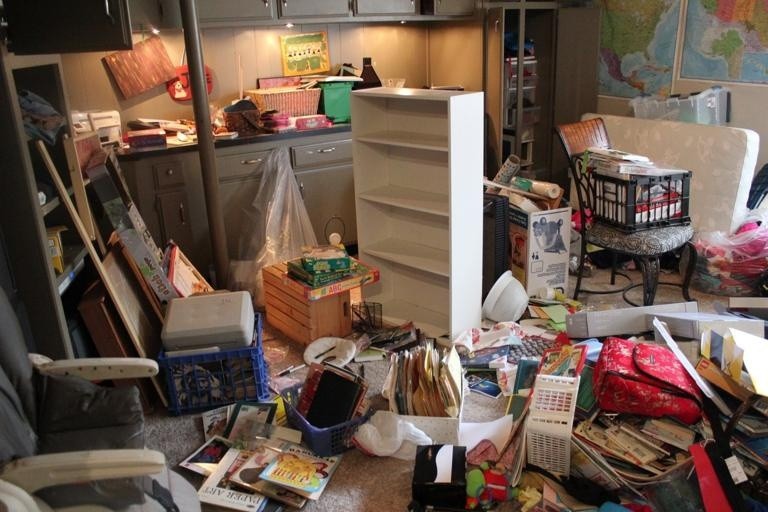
top-left (353, 56), bottom-right (382, 89)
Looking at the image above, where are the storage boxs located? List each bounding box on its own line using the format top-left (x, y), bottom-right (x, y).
top-left (511, 207), bottom-right (571, 310)
top-left (263, 261), bottom-right (349, 348)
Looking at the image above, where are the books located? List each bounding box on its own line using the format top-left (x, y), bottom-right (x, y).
top-left (354, 323), bottom-right (435, 363)
top-left (125, 117), bottom-right (239, 141)
top-left (585, 140), bottom-right (690, 228)
top-left (381, 343), bottom-right (463, 417)
top-left (297, 361), bottom-right (369, 450)
top-left (573, 407), bottom-right (768, 492)
top-left (174, 401), bottom-right (342, 511)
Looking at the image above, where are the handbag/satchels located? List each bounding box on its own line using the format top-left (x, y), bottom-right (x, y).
top-left (592, 336), bottom-right (705, 425)
top-left (103, 34), bottom-right (213, 101)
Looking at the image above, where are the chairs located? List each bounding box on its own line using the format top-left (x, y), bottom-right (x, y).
top-left (0, 291), bottom-right (199, 512)
top-left (560, 118), bottom-right (697, 308)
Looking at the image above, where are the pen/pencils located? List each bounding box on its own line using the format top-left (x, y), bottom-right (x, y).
top-left (315, 345), bottom-right (336, 359)
top-left (370, 346), bottom-right (397, 354)
top-left (354, 304), bottom-right (373, 328)
top-left (276, 363), bottom-right (306, 376)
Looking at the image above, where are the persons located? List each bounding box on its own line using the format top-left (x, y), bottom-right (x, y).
top-left (203, 445), bottom-right (226, 459)
top-left (206, 417), bottom-right (220, 434)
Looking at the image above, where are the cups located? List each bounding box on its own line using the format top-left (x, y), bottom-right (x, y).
top-left (538, 287), bottom-right (556, 300)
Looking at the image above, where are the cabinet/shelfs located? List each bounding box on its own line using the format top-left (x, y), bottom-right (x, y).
top-left (350, 87), bottom-right (483, 346)
top-left (0, 55), bottom-right (102, 386)
top-left (289, 133), bottom-right (355, 252)
top-left (215, 141), bottom-right (289, 262)
top-left (124, 154), bottom-right (212, 286)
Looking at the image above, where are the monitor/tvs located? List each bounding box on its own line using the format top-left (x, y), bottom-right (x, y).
top-left (3, 0), bottom-right (134, 56)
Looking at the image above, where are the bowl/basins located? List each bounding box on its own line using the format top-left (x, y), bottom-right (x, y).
top-left (481, 270), bottom-right (530, 325)
